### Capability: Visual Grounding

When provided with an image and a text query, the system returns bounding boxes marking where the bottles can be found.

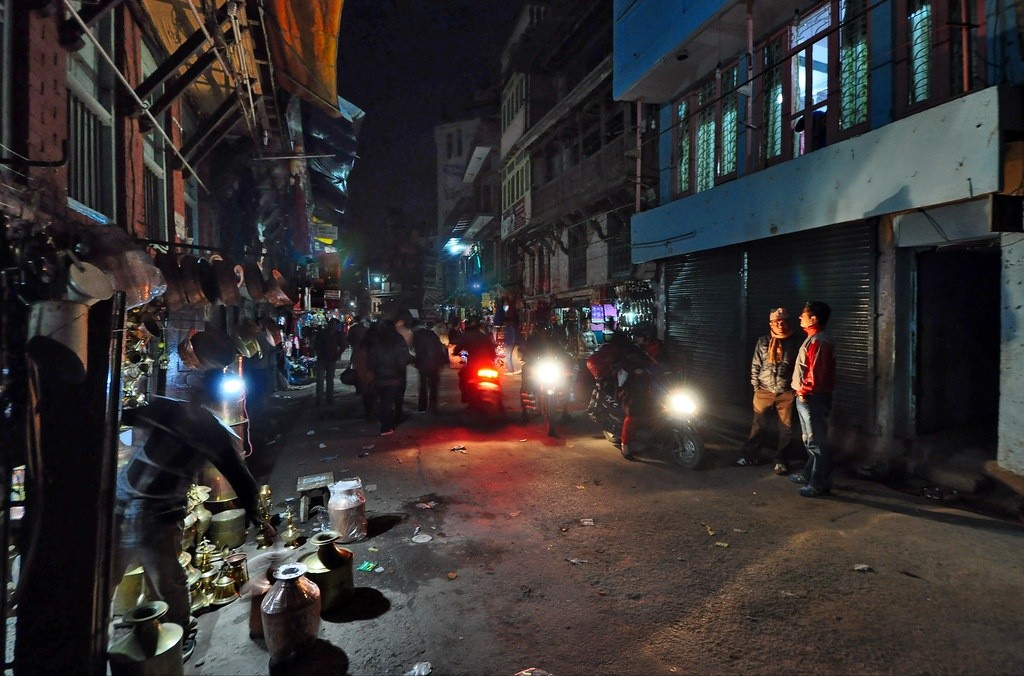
[108,478,365,676]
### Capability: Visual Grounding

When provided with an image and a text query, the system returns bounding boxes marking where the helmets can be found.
[631,321,657,344]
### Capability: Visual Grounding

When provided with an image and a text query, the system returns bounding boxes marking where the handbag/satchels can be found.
[340,360,357,384]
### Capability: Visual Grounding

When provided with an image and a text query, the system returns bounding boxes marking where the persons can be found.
[517,321,577,451]
[425,306,518,415]
[314,316,348,406]
[499,317,522,374]
[110,365,275,665]
[789,299,838,497]
[412,320,448,421]
[737,307,803,474]
[613,318,676,461]
[576,317,605,383]
[345,312,413,445]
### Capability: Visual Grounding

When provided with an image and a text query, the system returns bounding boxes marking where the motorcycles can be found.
[518,342,578,437]
[588,359,704,470]
[453,354,499,431]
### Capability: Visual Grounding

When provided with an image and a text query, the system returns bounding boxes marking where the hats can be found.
[770,307,789,320]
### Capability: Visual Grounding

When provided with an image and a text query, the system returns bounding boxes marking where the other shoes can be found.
[380,429,393,436]
[418,410,426,414]
[182,639,194,661]
[789,473,809,484]
[621,442,633,460]
[737,456,758,466]
[797,485,830,496]
[774,463,787,474]
